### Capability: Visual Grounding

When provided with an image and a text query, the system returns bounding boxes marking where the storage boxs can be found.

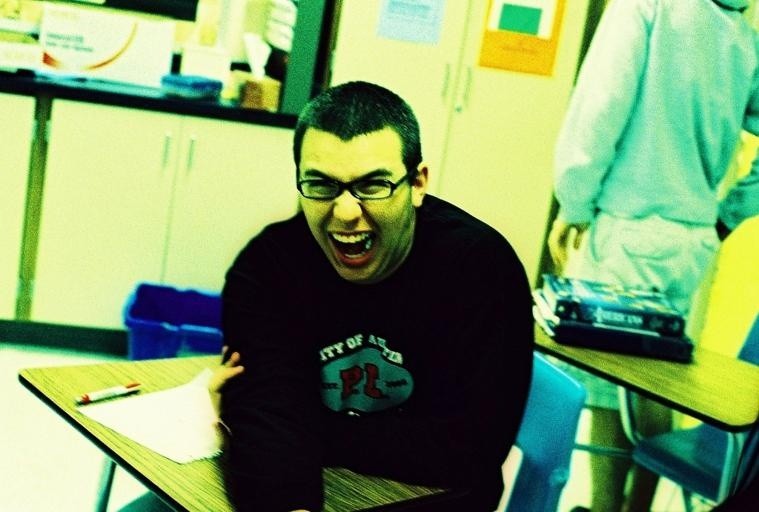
[122,280,224,361]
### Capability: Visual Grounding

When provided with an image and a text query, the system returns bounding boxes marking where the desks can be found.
[532,328,759,433]
[19,353,468,512]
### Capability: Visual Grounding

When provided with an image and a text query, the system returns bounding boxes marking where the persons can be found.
[540,1,759,512]
[118,76,538,512]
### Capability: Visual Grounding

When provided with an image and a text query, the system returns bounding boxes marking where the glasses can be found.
[296,167,410,201]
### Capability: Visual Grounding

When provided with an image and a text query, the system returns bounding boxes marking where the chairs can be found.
[498,349,587,512]
[620,312,759,512]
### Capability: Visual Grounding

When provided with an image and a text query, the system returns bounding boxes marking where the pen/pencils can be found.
[75,384,140,404]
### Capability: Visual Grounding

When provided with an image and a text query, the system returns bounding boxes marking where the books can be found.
[535,272,694,367]
